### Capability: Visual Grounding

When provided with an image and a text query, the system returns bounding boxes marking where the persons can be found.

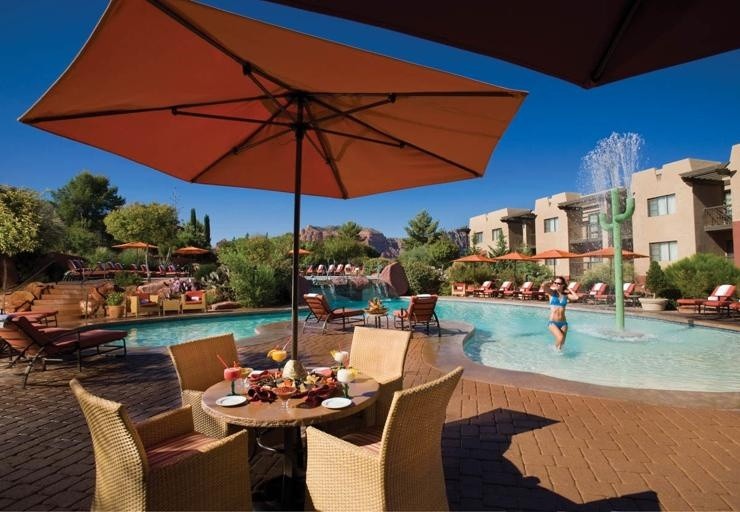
[180,280,192,301]
[540,276,580,351]
[169,276,181,295]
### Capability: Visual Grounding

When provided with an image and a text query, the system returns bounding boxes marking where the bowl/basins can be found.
[363,308,390,314]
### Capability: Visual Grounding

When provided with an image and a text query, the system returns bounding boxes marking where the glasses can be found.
[554,282,564,285]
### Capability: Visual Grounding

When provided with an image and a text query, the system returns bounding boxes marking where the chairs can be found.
[701,284,737,318]
[167,332,256,461]
[451,281,468,297]
[314,325,412,438]
[392,294,441,337]
[305,263,351,276]
[472,281,635,307]
[124,289,208,319]
[302,293,365,336]
[68,378,254,511]
[302,365,464,511]
[0,309,126,388]
[62,260,189,282]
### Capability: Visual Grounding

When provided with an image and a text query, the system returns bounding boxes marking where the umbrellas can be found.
[450,253,499,282]
[574,246,650,284]
[491,251,541,282]
[530,248,582,280]
[111,241,159,269]
[266,0,740,90]
[15,0,530,360]
[176,245,209,263]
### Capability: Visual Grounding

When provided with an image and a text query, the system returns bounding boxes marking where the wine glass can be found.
[238,366,251,395]
[271,351,288,373]
[273,387,297,413]
[333,350,350,367]
[223,367,241,395]
[337,369,354,399]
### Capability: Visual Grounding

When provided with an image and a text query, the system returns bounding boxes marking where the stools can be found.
[676,298,706,314]
[728,301,740,322]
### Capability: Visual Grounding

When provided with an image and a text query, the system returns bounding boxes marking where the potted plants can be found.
[105,291,125,319]
[206,289,223,309]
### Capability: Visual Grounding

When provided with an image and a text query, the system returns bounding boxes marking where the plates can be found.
[322,397,353,409]
[216,395,247,407]
